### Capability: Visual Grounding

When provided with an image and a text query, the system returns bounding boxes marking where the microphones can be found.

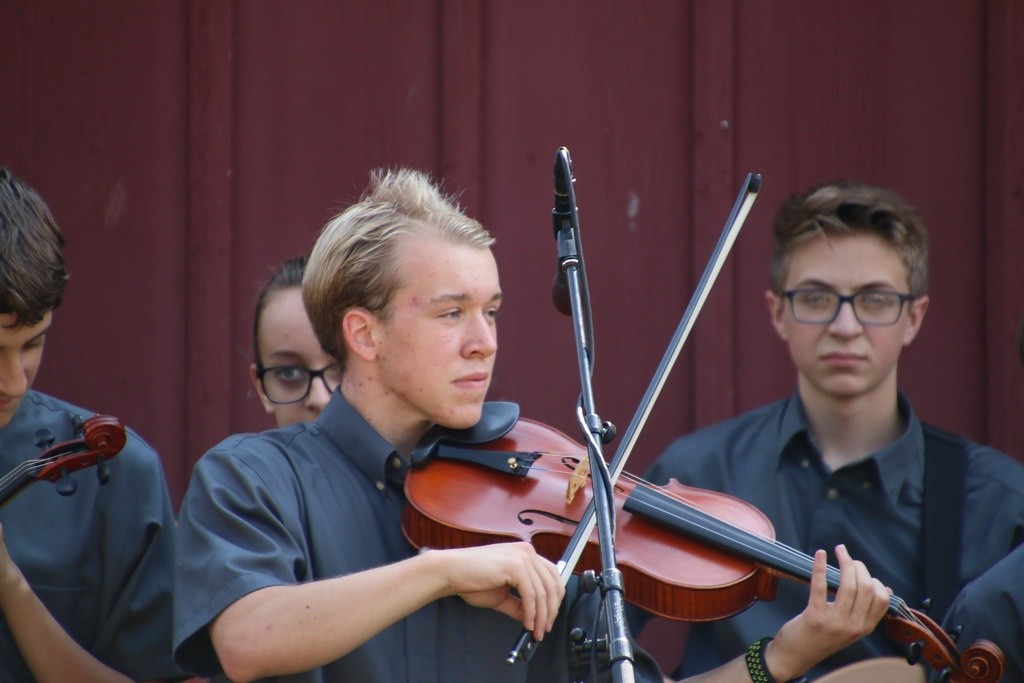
[552,148,590,315]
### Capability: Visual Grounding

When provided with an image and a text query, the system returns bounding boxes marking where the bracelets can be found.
[747,636,807,682]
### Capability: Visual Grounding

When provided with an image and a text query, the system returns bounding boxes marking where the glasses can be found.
[776,288,916,325]
[256,359,347,404]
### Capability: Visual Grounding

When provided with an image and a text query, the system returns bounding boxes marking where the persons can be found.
[169,167,894,683]
[0,170,174,683]
[616,187,1024,683]
[249,252,342,431]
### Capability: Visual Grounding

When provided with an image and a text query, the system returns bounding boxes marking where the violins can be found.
[399,413,1007,683]
[0,414,130,511]
